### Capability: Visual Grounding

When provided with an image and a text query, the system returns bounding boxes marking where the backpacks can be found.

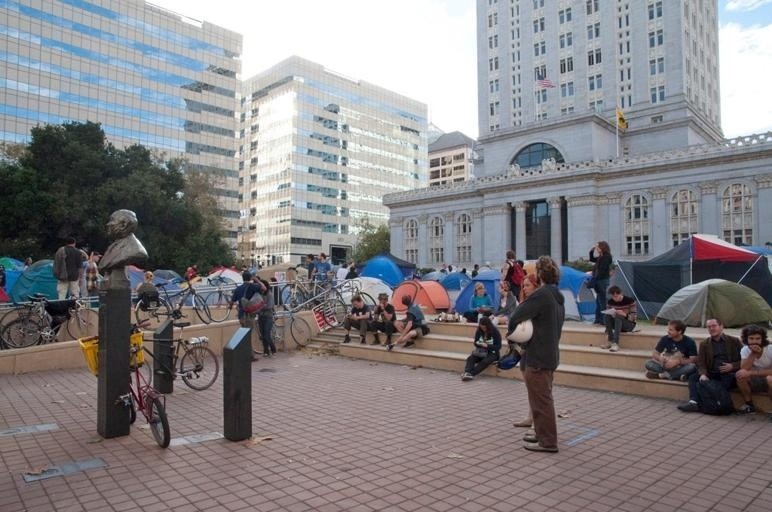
[696,377,737,416]
[53,246,68,281]
[508,258,525,285]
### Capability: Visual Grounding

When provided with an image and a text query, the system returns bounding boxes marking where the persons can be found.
[676,316,738,415]
[642,317,697,380]
[733,323,772,414]
[503,253,567,455]
[458,314,503,382]
[94,207,152,271]
[510,272,542,434]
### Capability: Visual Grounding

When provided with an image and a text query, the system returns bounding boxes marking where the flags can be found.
[536,72,556,91]
[615,107,630,131]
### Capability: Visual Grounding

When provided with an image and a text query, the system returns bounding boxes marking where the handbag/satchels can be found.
[471,345,489,358]
[240,284,266,313]
[422,323,430,335]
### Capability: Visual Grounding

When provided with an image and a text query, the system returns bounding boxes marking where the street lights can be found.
[241,253,262,268]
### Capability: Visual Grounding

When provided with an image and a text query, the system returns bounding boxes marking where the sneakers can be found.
[385,343,394,351]
[252,353,278,362]
[599,342,619,351]
[343,336,351,344]
[679,375,690,381]
[360,337,366,344]
[402,341,416,349]
[460,371,474,381]
[735,401,757,415]
[525,429,536,435]
[522,435,538,443]
[646,371,661,379]
[513,419,532,427]
[523,441,559,452]
[369,339,381,345]
[676,402,700,412]
[382,339,391,346]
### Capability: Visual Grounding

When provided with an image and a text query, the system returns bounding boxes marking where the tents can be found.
[607,232,772,321]
[651,278,772,331]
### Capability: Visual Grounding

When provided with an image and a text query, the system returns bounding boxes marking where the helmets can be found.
[505,318,534,343]
[496,347,523,371]
